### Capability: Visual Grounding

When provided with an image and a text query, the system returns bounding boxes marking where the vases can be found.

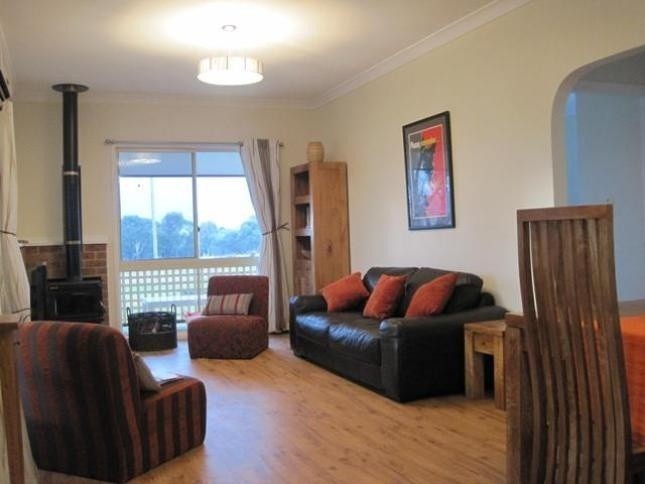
[307,142,324,163]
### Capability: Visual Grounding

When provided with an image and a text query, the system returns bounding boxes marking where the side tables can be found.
[463,319,507,409]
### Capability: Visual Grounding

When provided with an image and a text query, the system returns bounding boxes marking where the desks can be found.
[506,314,645,483]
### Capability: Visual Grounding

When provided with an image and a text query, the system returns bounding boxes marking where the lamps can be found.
[196,24,263,86]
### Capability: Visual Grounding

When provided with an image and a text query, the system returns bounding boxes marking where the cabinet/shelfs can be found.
[289,161,350,296]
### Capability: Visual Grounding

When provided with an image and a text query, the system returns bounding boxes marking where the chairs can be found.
[188,276,268,359]
[516,205,631,483]
[14,321,206,484]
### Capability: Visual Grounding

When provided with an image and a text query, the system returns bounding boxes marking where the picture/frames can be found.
[402,111,455,230]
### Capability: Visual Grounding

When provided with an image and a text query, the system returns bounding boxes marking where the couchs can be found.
[290,267,507,405]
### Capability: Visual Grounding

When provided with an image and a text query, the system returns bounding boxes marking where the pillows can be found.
[201,293,253,316]
[364,274,407,319]
[319,272,370,313]
[404,273,457,317]
[131,352,159,394]
